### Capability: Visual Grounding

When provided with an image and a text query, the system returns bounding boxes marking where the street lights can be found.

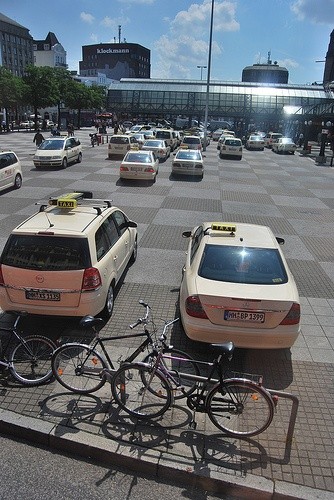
[197,66,207,80]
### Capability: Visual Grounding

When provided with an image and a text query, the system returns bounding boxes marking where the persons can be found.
[285,126,304,147]
[2,118,126,147]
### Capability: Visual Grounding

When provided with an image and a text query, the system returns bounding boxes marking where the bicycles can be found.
[0,309,60,387]
[50,299,200,400]
[113,318,278,438]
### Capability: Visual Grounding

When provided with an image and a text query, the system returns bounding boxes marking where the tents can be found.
[95,113,112,117]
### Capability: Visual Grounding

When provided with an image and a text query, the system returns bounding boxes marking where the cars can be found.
[0,150,23,191]
[195,128,283,151]
[272,138,296,154]
[123,120,183,152]
[9,120,58,129]
[180,136,203,153]
[32,135,83,170]
[219,138,243,159]
[142,140,171,161]
[0,190,138,318]
[120,147,159,183]
[178,221,301,349]
[172,149,206,178]
[108,134,139,159]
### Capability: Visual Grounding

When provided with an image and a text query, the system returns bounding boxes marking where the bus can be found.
[93,113,113,124]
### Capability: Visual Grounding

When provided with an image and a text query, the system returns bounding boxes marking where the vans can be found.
[176,119,232,130]
